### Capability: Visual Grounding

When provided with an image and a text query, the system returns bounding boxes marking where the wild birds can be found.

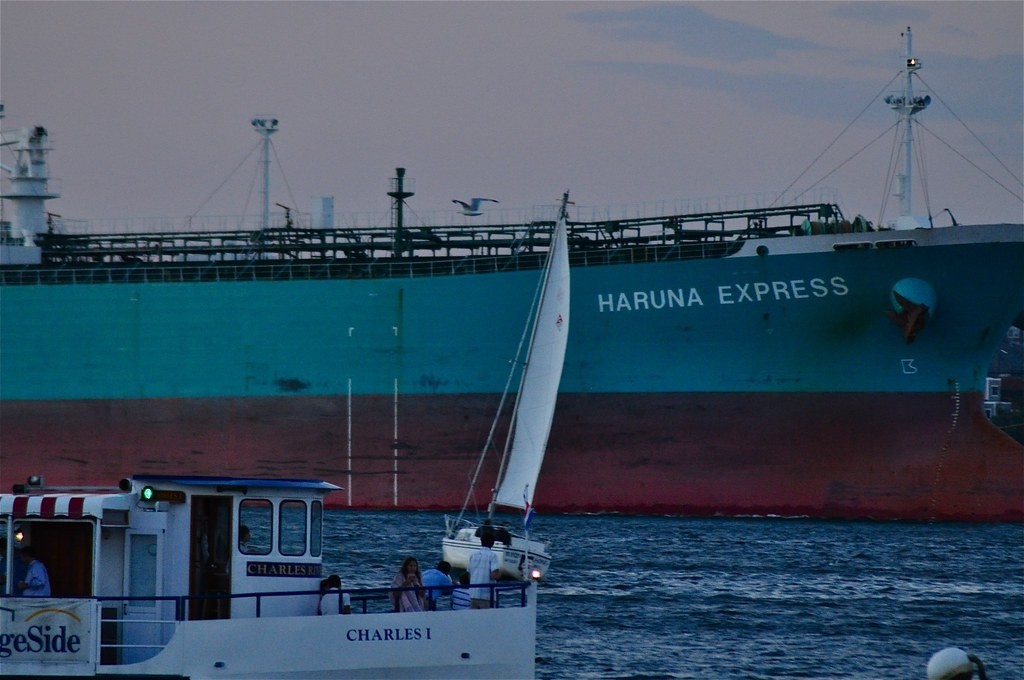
[452,197,501,216]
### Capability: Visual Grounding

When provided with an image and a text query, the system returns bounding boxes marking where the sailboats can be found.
[441,190,576,580]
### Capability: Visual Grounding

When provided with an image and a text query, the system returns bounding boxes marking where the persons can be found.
[420,561,453,611]
[226,524,255,573]
[451,572,471,611]
[317,575,351,616]
[0,537,52,596]
[387,557,425,613]
[466,531,501,609]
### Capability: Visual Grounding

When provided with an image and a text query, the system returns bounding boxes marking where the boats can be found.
[0,474,537,680]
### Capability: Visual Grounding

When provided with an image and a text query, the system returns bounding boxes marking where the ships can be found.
[0,24,1024,525]
